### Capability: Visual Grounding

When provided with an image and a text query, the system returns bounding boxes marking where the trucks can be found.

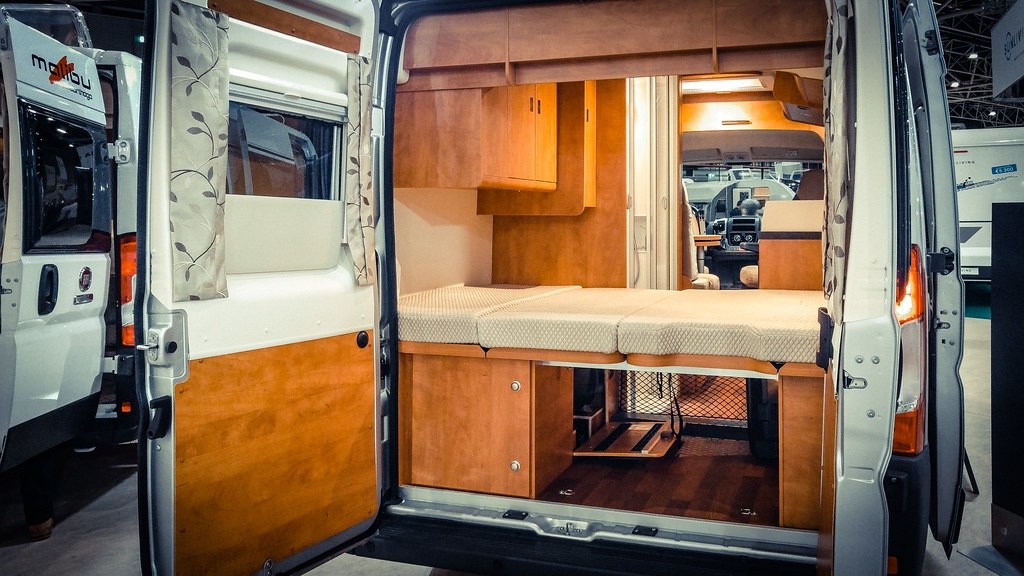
[1,8,320,476]
[127,1,965,576]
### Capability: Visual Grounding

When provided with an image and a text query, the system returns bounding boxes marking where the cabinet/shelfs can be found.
[398,354,573,499]
[393,83,557,192]
[477,80,596,217]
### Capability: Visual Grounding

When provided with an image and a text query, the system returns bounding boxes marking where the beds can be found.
[397,282,828,364]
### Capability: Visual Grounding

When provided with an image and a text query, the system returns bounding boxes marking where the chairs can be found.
[740,169,824,288]
[682,179,721,289]
